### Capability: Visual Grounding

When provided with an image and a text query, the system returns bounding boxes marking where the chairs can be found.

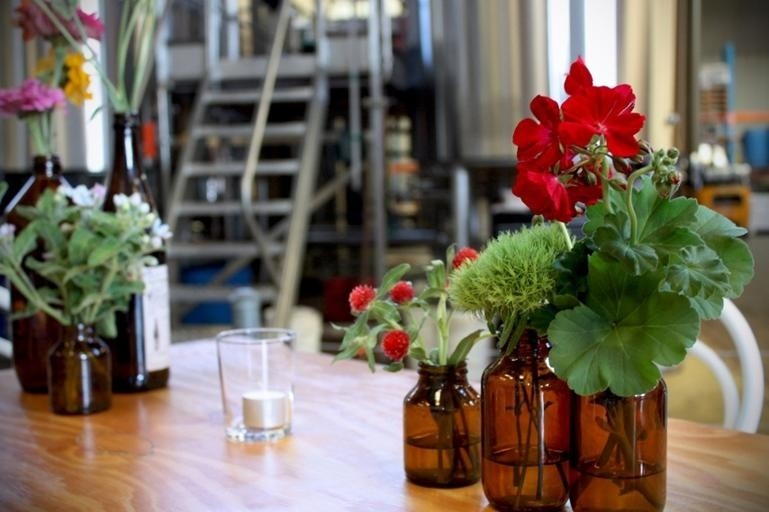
[657,292,765,432]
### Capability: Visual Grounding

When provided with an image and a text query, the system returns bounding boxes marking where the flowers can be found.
[338,244,493,472]
[1,177,176,398]
[450,224,566,497]
[512,61,757,505]
[1,1,105,155]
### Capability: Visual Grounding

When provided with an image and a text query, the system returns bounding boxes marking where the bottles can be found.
[571,376,669,510]
[47,323,114,416]
[404,359,483,487]
[101,112,174,390]
[4,154,83,398]
[483,327,570,512]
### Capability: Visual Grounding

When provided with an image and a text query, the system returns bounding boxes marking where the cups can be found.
[215,328,298,443]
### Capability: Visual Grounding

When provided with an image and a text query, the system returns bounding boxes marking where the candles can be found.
[243,391,287,428]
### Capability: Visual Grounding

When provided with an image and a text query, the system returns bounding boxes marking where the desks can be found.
[4,328,768,509]
